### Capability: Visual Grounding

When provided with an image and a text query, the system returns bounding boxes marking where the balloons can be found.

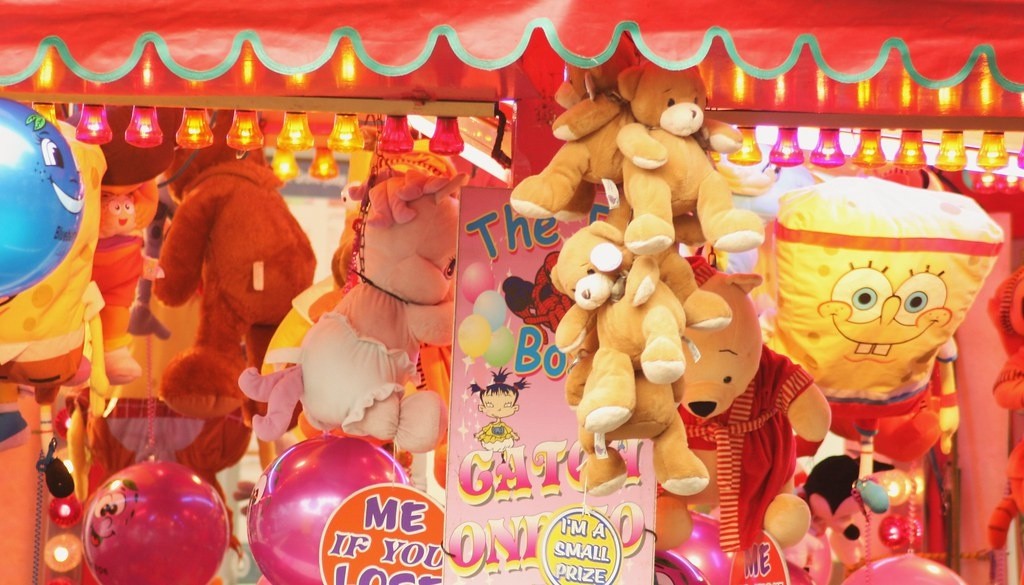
[83,458,229,585]
[246,436,407,585]
[0,97,81,298]
[652,512,733,584]
[838,554,965,585]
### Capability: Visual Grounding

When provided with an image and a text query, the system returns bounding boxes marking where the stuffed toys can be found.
[774,177,1003,458]
[795,456,895,540]
[988,266,1024,552]
[0,107,471,550]
[512,30,763,495]
[656,267,832,542]
